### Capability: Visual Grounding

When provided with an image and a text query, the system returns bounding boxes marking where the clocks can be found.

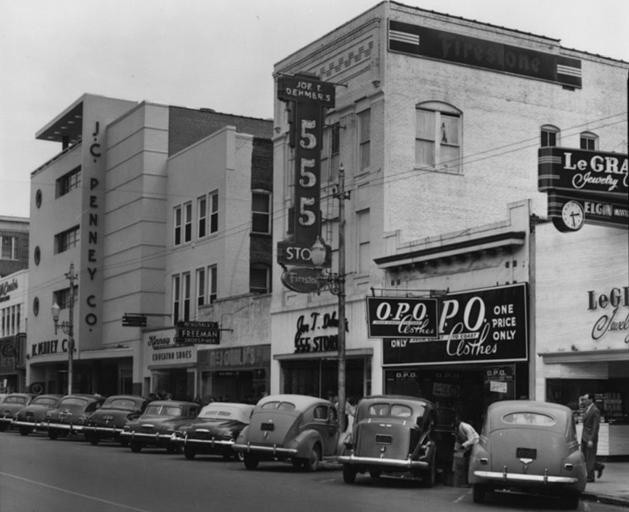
[554,200,585,233]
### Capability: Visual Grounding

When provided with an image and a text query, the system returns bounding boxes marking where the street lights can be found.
[309,232,350,435]
[50,302,74,395]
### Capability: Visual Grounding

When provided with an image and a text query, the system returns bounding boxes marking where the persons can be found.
[579,393,605,483]
[330,395,357,435]
[140,389,173,413]
[445,415,479,488]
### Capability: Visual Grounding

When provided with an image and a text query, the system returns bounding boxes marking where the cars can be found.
[121,397,205,455]
[183,400,257,461]
[0,388,144,449]
[231,394,344,470]
[336,393,456,485]
[469,398,587,507]
